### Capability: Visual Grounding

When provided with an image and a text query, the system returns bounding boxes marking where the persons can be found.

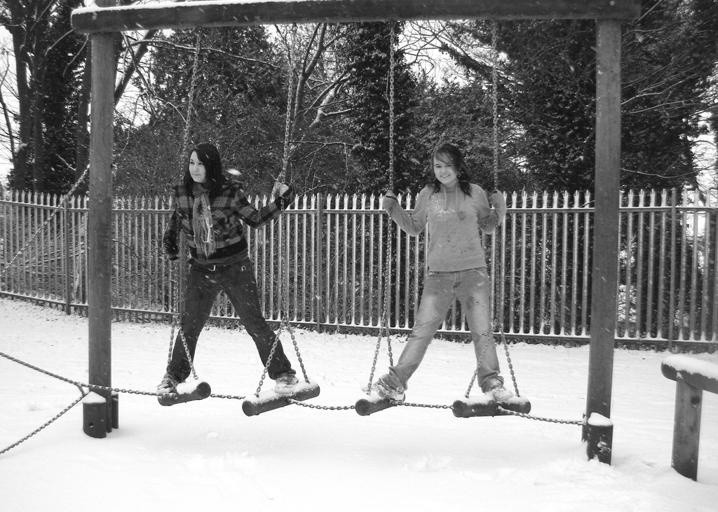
[155,142,299,394]
[373,142,514,402]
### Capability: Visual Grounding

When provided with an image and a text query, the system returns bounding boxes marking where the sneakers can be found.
[361,379,407,404]
[274,372,299,396]
[484,384,516,404]
[155,373,184,395]
